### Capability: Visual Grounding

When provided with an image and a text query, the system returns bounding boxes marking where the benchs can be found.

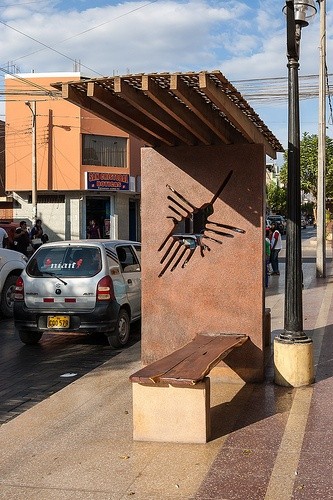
[131,333,247,444]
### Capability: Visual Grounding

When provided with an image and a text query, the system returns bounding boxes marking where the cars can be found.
[301,216,308,228]
[14,239,143,348]
[265,214,287,236]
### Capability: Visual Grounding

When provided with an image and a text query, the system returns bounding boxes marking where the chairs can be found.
[67,251,93,272]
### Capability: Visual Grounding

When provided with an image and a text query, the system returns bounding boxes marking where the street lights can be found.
[272,0,319,388]
[25,99,38,227]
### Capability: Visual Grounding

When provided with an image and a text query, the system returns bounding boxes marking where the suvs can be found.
[0,249,28,319]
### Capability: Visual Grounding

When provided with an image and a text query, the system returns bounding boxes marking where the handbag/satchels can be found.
[31,235,43,247]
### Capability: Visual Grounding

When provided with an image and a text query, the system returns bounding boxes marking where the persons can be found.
[13,221,30,258]
[87,219,101,239]
[265,219,287,288]
[0,227,8,248]
[31,219,47,255]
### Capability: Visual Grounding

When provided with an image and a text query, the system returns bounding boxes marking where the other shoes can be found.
[271,271,280,275]
